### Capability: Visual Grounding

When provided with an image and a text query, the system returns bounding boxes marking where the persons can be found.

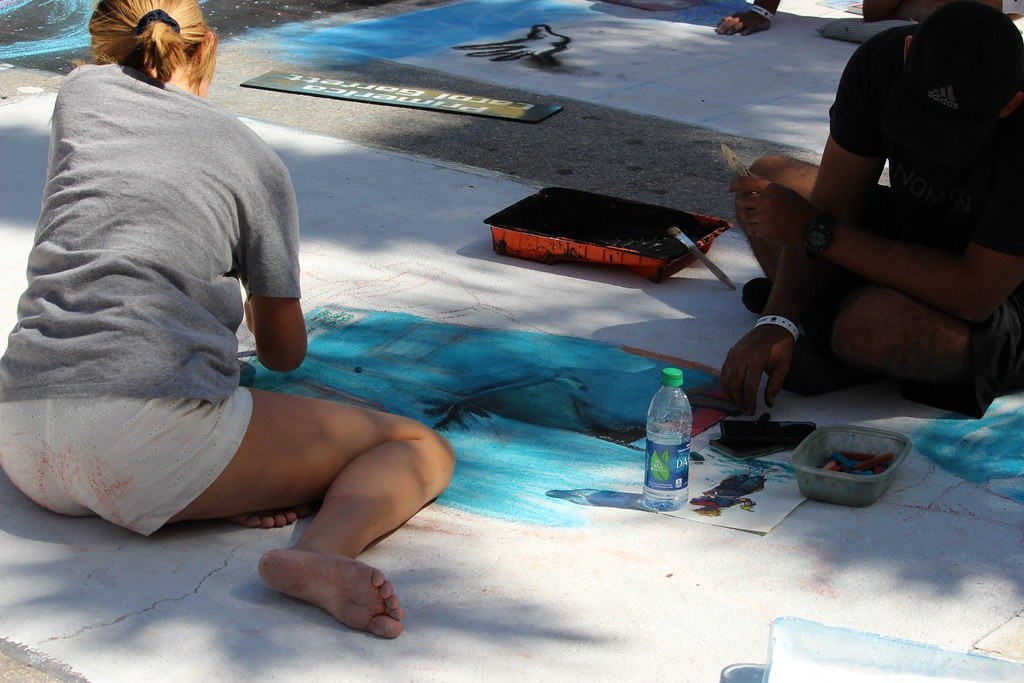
[1,0,459,641]
[714,0,1024,35]
[718,0,1024,419]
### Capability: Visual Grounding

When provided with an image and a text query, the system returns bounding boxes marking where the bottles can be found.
[643,367,693,511]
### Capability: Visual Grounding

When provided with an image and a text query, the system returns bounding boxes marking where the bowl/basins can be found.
[791,424,911,507]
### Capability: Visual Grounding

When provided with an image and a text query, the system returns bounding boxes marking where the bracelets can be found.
[755,313,800,344]
[751,4,776,22]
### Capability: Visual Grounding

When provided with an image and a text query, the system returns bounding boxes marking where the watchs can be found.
[801,210,838,259]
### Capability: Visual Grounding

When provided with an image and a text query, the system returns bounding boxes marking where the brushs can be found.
[668,225,737,288]
